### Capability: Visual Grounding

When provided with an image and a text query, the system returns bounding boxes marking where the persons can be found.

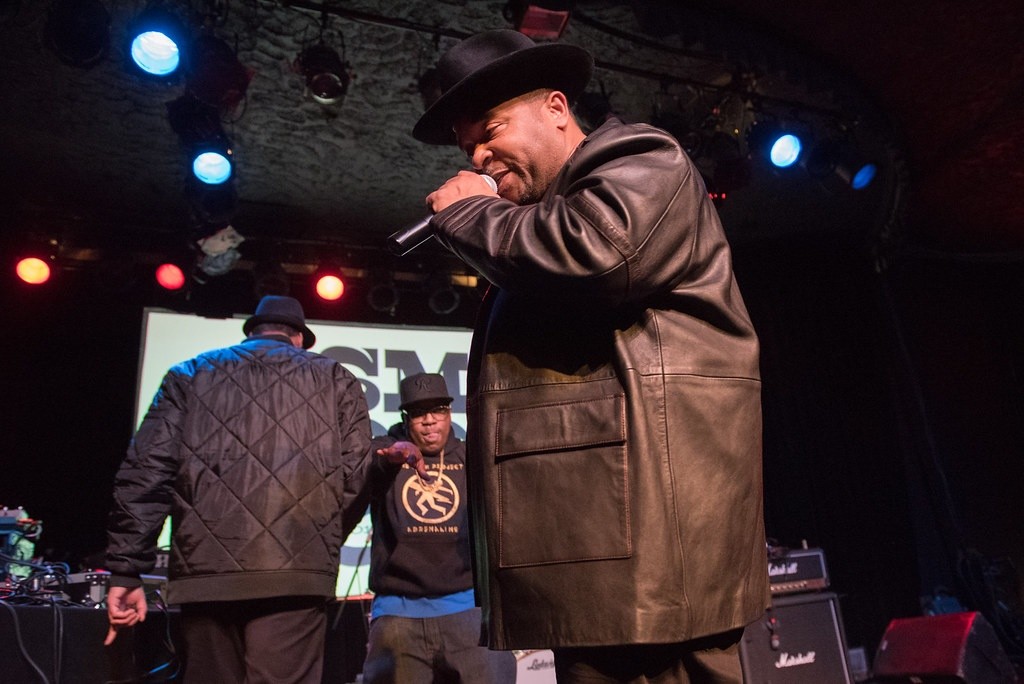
[98,296,376,683]
[426,39,770,684]
[360,381,519,681]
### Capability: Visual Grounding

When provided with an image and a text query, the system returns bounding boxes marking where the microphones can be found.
[366,528,373,543]
[387,174,498,255]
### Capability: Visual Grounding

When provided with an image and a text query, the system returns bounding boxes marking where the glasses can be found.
[402,402,452,424]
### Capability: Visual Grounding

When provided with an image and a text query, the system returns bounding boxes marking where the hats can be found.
[398,372,454,411]
[411,29,594,147]
[242,295,317,350]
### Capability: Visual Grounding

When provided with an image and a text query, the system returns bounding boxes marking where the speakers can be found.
[735,590,1022,684]
